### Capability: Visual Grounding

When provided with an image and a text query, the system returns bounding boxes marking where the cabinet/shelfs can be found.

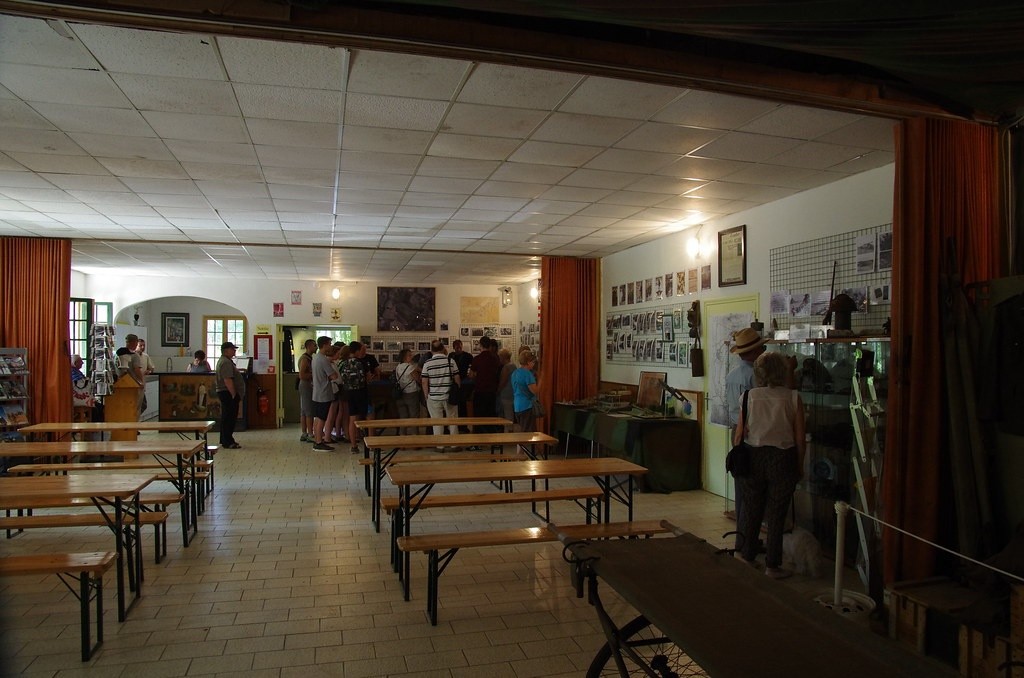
[764,337,890,572]
[0,347,34,477]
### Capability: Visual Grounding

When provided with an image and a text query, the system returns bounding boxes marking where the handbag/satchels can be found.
[447,383,459,405]
[295,377,300,389]
[726,445,750,479]
[532,400,545,417]
[72,379,96,408]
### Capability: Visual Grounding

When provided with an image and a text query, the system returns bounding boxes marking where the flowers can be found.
[134,304,144,314]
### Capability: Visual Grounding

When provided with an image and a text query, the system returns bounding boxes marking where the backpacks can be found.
[392,365,415,399]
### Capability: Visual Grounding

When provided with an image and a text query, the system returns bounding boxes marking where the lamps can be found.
[331,284,343,300]
[499,287,515,306]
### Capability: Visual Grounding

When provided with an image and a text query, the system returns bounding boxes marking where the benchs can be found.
[0,511,169,592]
[7,459,218,515]
[379,488,604,571]
[156,472,209,528]
[0,552,118,662]
[0,493,185,565]
[395,520,675,626]
[357,454,529,493]
[154,472,210,517]
[205,442,222,491]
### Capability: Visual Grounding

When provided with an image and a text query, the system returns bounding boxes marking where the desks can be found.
[364,432,558,532]
[0,439,204,549]
[0,474,159,622]
[597,407,701,493]
[552,398,632,459]
[354,417,512,496]
[384,457,648,602]
[16,421,217,494]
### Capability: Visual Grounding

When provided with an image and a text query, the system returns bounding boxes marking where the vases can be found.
[134,314,140,325]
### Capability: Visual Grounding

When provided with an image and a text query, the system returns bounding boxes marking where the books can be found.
[0,355,31,476]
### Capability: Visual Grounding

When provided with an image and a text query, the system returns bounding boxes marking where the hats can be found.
[221,342,239,349]
[729,328,770,354]
[126,334,141,342]
[116,348,136,355]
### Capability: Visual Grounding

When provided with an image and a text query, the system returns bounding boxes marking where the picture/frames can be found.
[359,286,540,369]
[668,343,677,366]
[606,223,747,369]
[636,371,666,410]
[159,311,190,348]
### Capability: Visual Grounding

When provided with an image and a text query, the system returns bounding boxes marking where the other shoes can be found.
[495,446,500,449]
[222,442,241,449]
[435,448,445,453]
[452,447,463,452]
[766,566,791,579]
[760,547,768,553]
[300,432,362,453]
[466,446,483,451]
[743,559,760,568]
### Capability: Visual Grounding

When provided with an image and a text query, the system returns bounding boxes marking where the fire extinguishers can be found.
[255,383,271,416]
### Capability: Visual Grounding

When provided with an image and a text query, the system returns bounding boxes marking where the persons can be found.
[187,350,211,373]
[114,334,143,382]
[134,339,155,435]
[727,327,771,551]
[215,342,245,449]
[735,352,807,579]
[71,355,104,424]
[298,335,541,462]
